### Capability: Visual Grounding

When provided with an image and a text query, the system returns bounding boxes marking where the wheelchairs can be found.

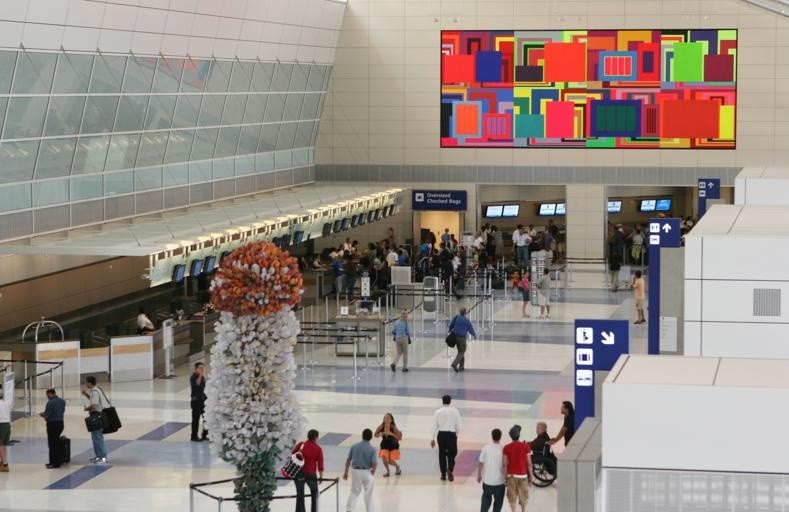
[529,439,559,487]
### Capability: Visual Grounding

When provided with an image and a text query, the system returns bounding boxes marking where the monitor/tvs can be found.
[323,204,397,238]
[608,200,622,213]
[486,204,520,218]
[173,231,305,283]
[539,203,566,216]
[640,199,672,212]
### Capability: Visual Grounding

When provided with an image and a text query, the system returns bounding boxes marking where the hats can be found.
[509,425,522,438]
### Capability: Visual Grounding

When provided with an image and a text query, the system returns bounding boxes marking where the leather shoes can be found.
[45,463,61,469]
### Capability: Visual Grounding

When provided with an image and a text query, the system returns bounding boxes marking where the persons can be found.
[82,376,110,464]
[0,389,11,472]
[343,395,574,512]
[190,362,209,442]
[292,429,324,512]
[137,304,155,334]
[448,307,477,373]
[390,308,411,372]
[39,388,66,468]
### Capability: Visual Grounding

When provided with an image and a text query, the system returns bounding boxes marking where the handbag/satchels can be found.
[277,440,305,487]
[84,410,110,433]
[542,443,558,476]
[408,335,412,344]
[97,406,123,435]
[445,331,459,349]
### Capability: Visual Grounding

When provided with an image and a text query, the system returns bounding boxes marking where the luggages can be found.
[58,435,72,465]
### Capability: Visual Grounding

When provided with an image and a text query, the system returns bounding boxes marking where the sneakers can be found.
[391,364,397,373]
[450,363,465,373]
[456,295,465,301]
[521,314,552,319]
[88,455,110,465]
[395,469,402,476]
[633,318,646,324]
[401,368,409,372]
[191,437,210,442]
[382,472,391,477]
[0,460,10,473]
[440,468,454,482]
